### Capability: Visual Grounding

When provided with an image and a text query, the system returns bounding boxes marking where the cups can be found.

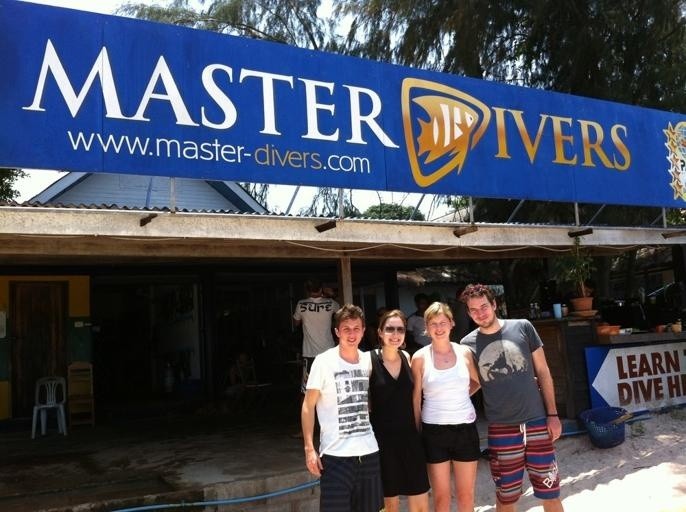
[673,324,681,333]
[553,304,562,318]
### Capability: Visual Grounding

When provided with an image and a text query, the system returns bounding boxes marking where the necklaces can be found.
[444,359,450,363]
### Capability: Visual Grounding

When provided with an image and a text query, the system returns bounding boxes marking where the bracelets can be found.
[547,412,559,417]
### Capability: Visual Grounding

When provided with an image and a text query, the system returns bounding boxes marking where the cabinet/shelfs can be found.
[532,315,601,421]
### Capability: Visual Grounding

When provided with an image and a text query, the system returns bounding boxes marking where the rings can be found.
[558,433,562,435]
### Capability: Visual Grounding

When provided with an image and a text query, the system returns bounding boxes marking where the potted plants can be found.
[547,249,598,316]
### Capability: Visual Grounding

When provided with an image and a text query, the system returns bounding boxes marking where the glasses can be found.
[381,326,406,334]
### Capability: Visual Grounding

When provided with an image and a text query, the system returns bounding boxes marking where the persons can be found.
[291,276,342,440]
[459,282,566,512]
[411,301,543,511]
[300,303,385,512]
[406,294,433,353]
[363,309,432,512]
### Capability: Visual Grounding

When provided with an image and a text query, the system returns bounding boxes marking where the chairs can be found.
[30,360,94,438]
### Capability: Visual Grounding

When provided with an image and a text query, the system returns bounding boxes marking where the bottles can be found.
[676,319,682,332]
[562,304,569,317]
[530,303,549,318]
